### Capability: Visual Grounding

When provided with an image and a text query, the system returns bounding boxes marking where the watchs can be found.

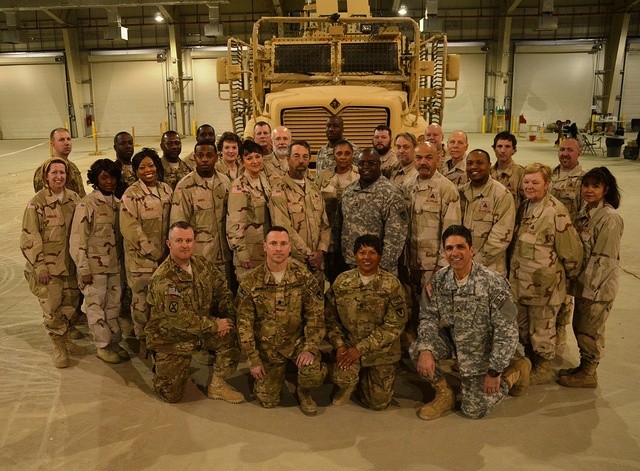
[488,368,501,377]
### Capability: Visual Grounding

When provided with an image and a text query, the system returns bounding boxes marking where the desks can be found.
[601,135,627,157]
[493,114,515,133]
[591,114,626,134]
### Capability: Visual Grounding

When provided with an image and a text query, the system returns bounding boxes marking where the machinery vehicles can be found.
[216,0,460,177]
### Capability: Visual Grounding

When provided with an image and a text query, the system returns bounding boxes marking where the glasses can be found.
[357,160,380,166]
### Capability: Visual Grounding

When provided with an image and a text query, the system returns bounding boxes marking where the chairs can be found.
[583,135,597,156]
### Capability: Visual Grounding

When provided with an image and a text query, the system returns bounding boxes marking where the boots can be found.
[64,338,85,355]
[54,337,68,368]
[419,378,455,420]
[501,357,532,397]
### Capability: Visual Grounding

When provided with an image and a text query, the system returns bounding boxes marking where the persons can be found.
[424,122,449,175]
[315,140,360,285]
[119,147,173,359]
[385,131,418,187]
[507,162,584,385]
[69,158,129,364]
[452,148,516,372]
[182,124,220,171]
[267,140,331,292]
[160,130,193,190]
[254,121,272,155]
[34,127,87,326]
[402,140,461,323]
[338,149,410,278]
[114,131,137,198]
[214,132,244,180]
[316,115,361,179]
[225,141,272,283]
[489,131,525,210]
[262,125,316,189]
[372,124,398,167]
[19,156,82,369]
[324,234,408,411]
[234,226,328,416]
[436,128,471,187]
[169,139,231,355]
[546,138,587,348]
[408,224,532,420]
[558,166,624,388]
[144,221,245,404]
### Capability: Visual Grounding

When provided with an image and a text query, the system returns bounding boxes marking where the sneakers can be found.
[558,367,598,380]
[297,386,317,416]
[332,386,353,406]
[559,372,597,388]
[208,383,244,404]
[110,343,130,361]
[119,315,135,337]
[529,355,554,376]
[193,349,214,366]
[71,310,87,325]
[139,340,148,358]
[97,347,120,364]
[66,327,82,339]
